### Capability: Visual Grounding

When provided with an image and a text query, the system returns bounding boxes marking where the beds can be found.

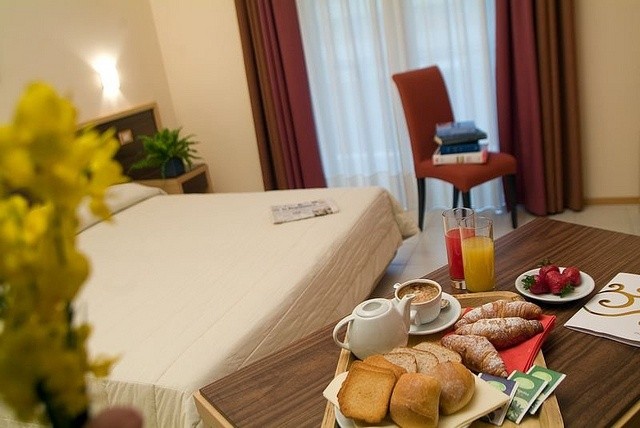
[69,182,421,428]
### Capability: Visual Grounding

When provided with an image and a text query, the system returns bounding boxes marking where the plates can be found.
[334,405,472,428]
[391,291,462,336]
[514,266,596,304]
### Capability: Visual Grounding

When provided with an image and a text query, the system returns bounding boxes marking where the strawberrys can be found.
[524,261,581,298]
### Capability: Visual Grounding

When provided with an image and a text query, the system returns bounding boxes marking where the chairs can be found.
[392,66,519,232]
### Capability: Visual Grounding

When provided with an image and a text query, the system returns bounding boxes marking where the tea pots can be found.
[332,294,416,360]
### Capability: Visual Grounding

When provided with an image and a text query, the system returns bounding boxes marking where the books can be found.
[431,121,489,165]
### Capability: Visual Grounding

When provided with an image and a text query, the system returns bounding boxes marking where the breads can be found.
[337,342,475,428]
[440,299,545,378]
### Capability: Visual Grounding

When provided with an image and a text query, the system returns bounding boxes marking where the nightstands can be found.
[75,102,213,194]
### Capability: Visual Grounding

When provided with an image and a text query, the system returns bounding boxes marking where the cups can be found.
[459,216,496,293]
[441,207,475,291]
[394,279,442,327]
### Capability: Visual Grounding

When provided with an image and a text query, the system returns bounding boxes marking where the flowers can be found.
[0,83,125,428]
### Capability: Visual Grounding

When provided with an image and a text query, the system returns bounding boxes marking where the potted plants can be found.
[127,127,203,178]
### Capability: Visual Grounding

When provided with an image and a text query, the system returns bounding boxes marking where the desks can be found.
[193,217,640,428]
